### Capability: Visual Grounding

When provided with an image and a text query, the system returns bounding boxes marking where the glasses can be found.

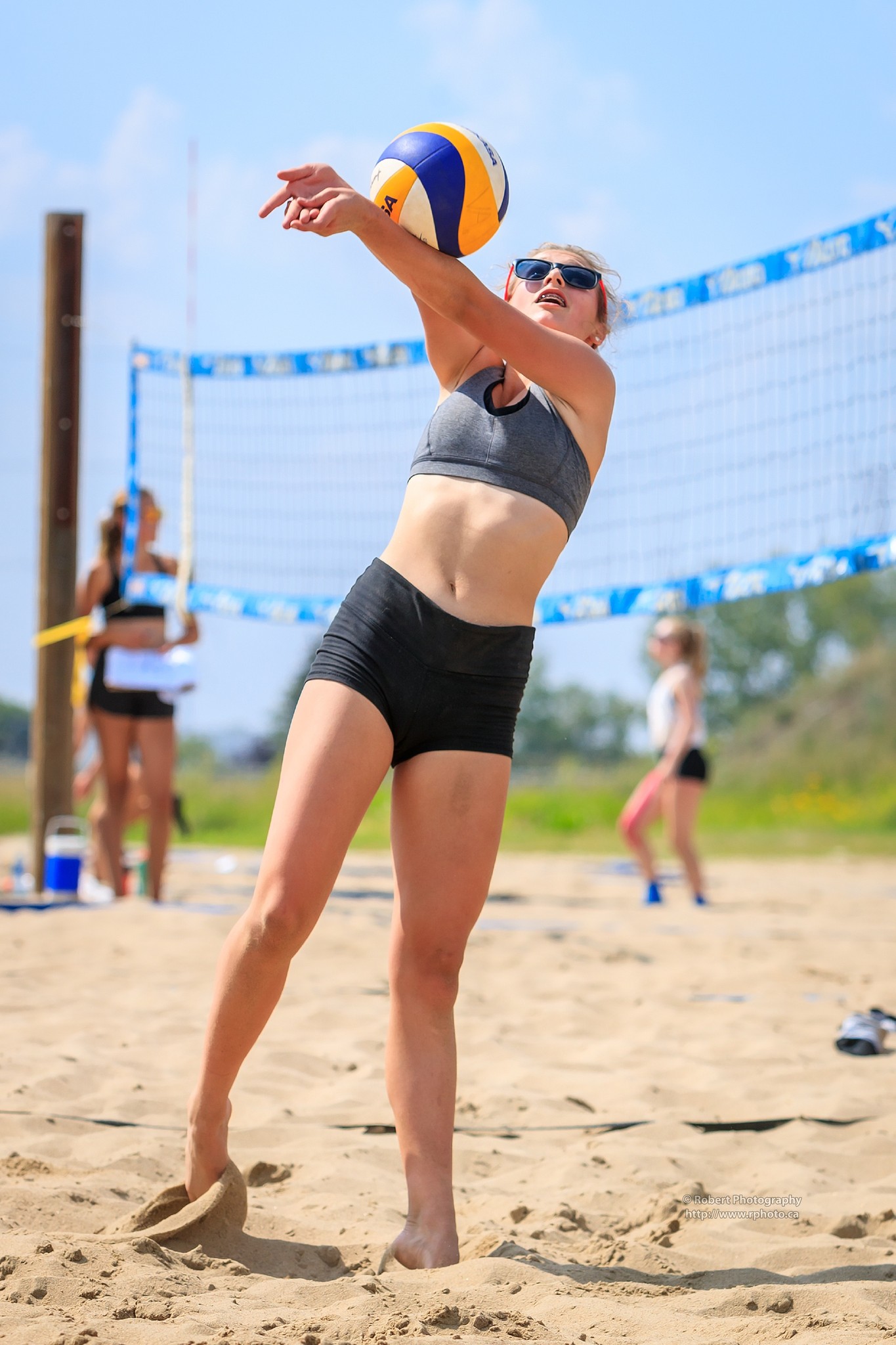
[504,258,607,322]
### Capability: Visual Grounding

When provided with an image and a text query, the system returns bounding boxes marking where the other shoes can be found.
[645,882,663,904]
[695,894,706,905]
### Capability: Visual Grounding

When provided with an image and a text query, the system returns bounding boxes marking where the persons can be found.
[181,162,629,1266]
[615,617,709,909]
[73,486,197,902]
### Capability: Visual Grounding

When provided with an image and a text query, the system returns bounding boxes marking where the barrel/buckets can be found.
[42,816,89,896]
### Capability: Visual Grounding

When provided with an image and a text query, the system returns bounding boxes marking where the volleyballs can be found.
[369,122,509,259]
